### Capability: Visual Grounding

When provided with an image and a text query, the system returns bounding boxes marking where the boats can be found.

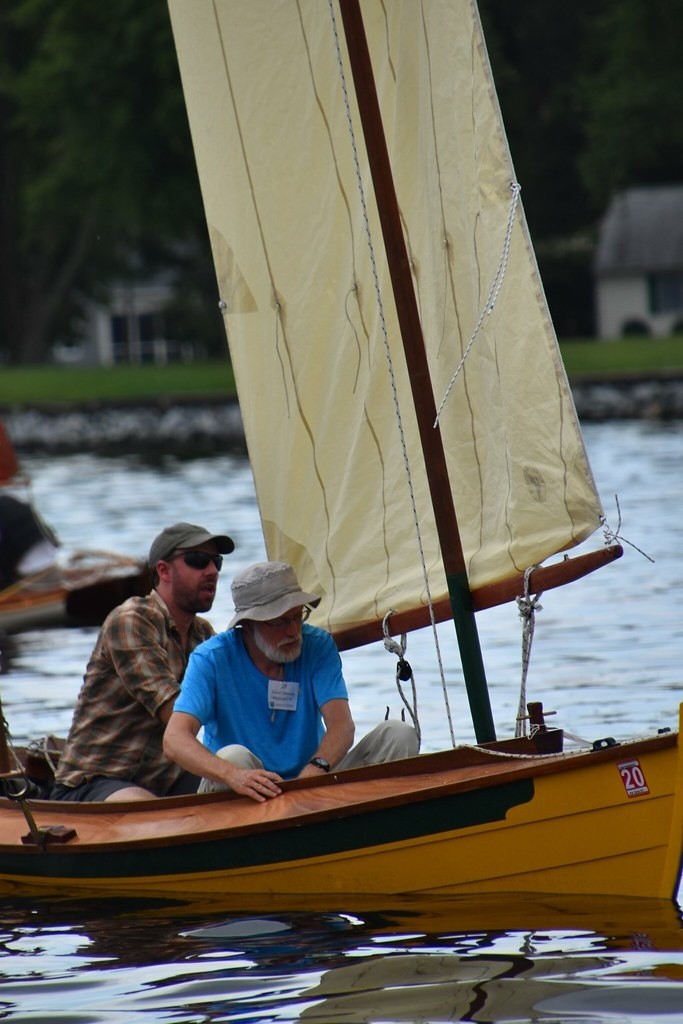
[1,549,162,638]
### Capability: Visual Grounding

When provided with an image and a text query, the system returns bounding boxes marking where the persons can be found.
[54,521,235,804]
[162,561,419,803]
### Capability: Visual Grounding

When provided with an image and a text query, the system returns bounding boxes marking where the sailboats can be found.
[0,0,682,915]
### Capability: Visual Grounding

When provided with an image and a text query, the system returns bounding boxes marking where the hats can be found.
[227,562,321,629]
[149,522,234,574]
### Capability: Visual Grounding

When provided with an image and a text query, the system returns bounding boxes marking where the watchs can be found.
[308,757,330,772]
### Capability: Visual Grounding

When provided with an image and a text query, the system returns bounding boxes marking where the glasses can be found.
[168,550,223,571]
[263,604,312,629]
